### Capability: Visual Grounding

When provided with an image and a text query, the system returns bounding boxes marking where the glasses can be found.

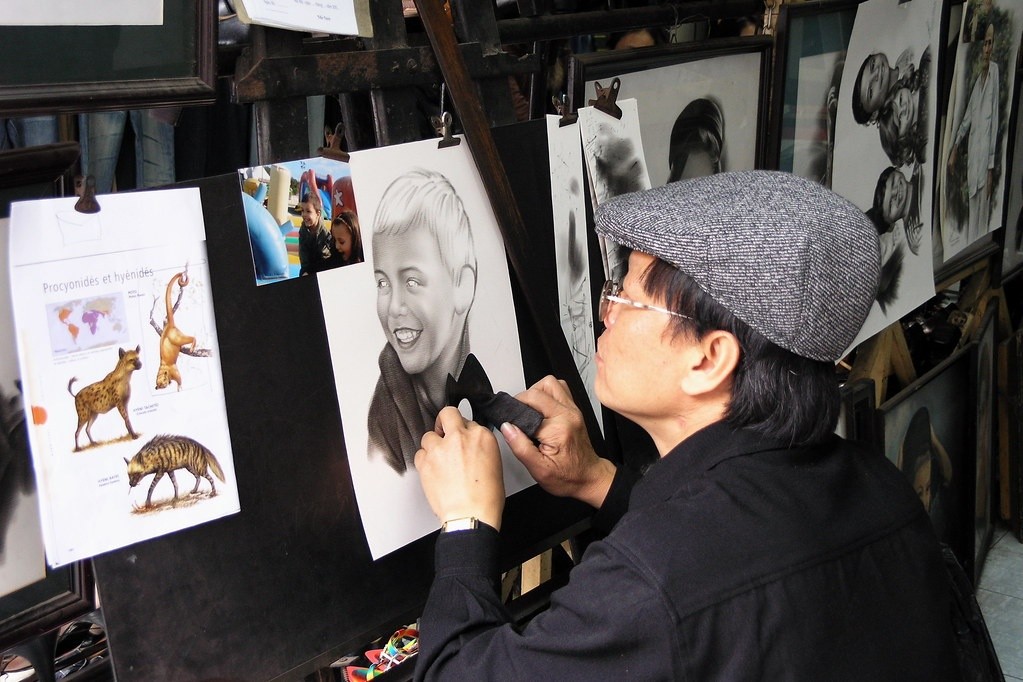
[600,280,693,322]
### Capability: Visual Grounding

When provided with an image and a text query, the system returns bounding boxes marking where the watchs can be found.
[441,517,499,538]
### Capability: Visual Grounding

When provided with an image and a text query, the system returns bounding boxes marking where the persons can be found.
[298,193,365,277]
[414,170,1006,682]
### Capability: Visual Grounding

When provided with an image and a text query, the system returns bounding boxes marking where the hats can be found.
[594,170,880,361]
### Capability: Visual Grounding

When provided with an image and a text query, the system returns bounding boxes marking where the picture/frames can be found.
[0,3,217,121]
[933,0,1010,287]
[562,37,772,188]
